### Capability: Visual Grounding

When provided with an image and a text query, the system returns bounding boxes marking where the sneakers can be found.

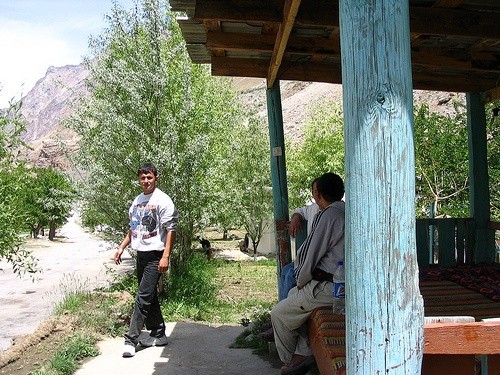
[122,345,135,356]
[139,335,169,347]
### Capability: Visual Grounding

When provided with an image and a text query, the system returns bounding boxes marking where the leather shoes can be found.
[257,321,275,341]
[281,354,317,375]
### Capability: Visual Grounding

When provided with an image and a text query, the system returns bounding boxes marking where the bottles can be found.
[333,261,346,316]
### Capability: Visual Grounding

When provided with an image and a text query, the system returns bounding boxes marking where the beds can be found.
[307,263,500,375]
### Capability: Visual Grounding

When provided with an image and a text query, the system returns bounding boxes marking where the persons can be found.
[270,172,346,375]
[114,161,179,358]
[290,178,324,238]
